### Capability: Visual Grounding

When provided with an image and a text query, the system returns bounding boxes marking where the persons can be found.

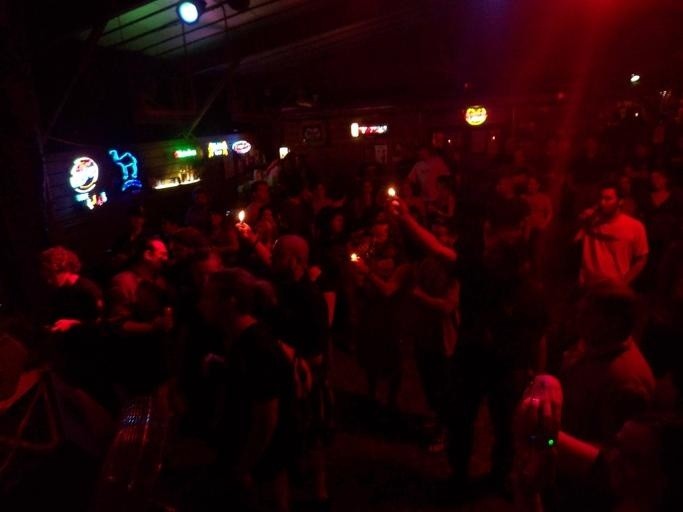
[1,122,683,512]
